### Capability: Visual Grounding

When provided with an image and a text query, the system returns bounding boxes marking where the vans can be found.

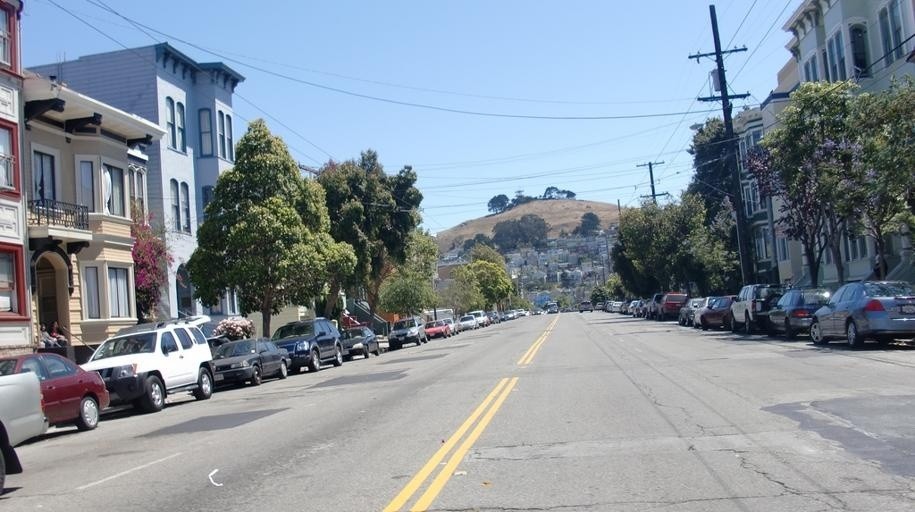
[546,302,558,314]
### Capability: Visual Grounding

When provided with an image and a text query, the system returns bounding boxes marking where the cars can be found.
[578,300,594,313]
[209,334,293,390]
[422,306,545,343]
[338,325,382,362]
[594,290,735,331]
[766,285,832,339]
[0,349,114,436]
[805,280,915,348]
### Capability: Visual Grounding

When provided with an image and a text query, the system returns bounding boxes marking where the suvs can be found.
[79,313,218,417]
[386,315,429,349]
[270,314,345,374]
[728,281,792,335]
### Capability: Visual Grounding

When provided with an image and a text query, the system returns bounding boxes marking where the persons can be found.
[875,251,883,281]
[48,320,68,346]
[39,319,58,348]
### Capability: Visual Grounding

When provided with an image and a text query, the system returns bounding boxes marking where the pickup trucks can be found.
[0,368,55,495]
